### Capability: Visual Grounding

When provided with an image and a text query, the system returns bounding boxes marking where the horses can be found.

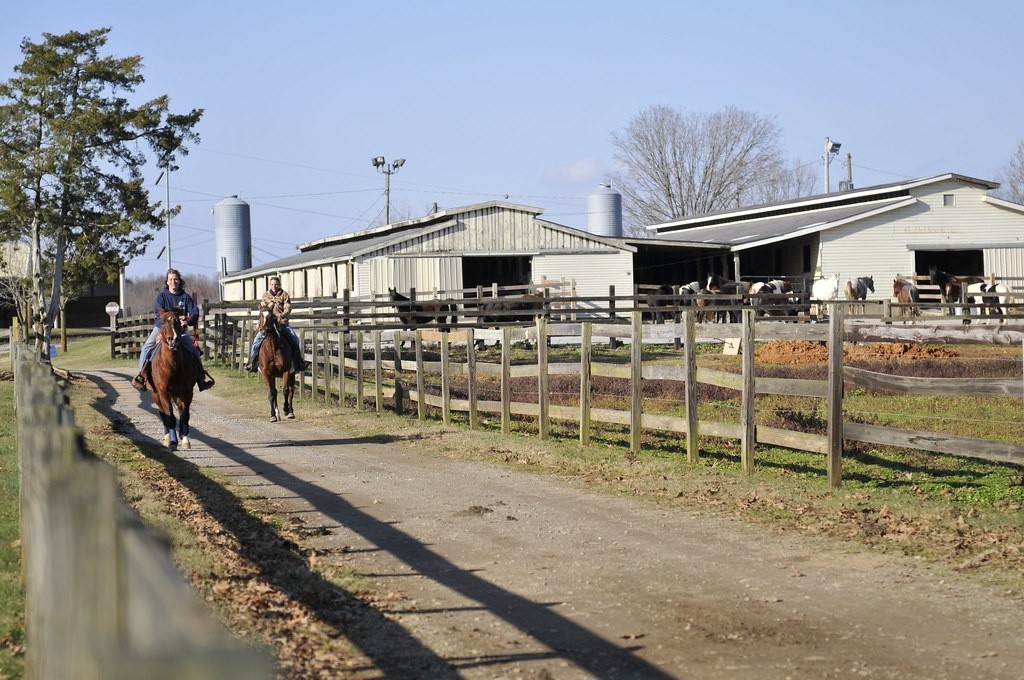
[148,306,196,451]
[811,264,1015,324]
[484,290,545,322]
[648,284,673,324]
[679,273,794,322]
[257,301,297,422]
[388,286,457,332]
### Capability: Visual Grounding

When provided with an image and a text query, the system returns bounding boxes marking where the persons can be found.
[245,277,308,371]
[132,269,215,392]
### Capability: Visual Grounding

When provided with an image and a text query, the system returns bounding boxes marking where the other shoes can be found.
[198,378,212,392]
[297,361,308,371]
[141,384,147,391]
[245,364,258,373]
